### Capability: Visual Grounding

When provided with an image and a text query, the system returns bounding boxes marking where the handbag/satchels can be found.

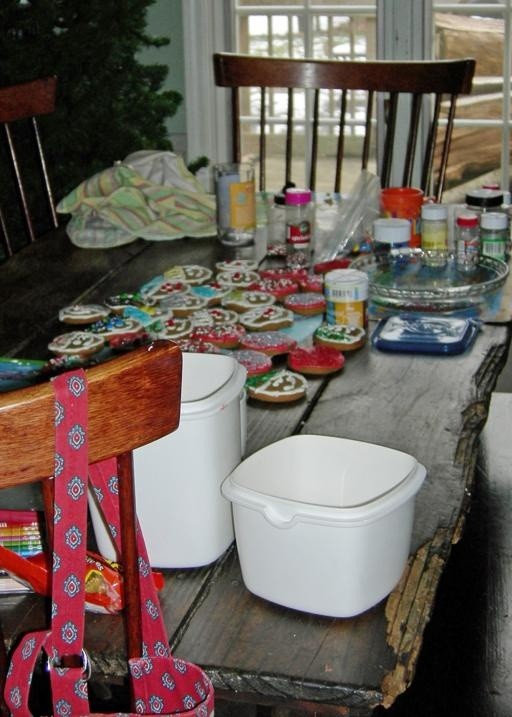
[3,368,218,717]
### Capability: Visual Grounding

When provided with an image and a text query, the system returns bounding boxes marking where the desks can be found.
[0,192,512,717]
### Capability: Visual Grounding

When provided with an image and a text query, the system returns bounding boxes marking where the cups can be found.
[209,160,257,249]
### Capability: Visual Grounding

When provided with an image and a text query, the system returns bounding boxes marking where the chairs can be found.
[0,340,183,717]
[0,73,59,257]
[211,51,476,205]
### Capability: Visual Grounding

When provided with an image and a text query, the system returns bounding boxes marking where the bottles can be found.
[321,266,371,338]
[371,187,511,261]
[264,185,315,256]
